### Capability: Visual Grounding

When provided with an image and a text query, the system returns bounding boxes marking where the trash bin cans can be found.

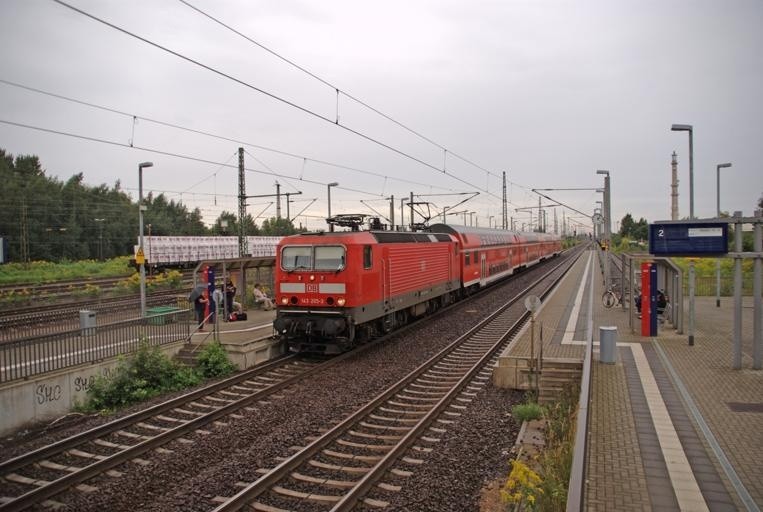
[79,310,96,337]
[599,327,617,365]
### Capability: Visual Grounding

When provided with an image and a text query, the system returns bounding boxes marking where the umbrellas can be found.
[189,284,206,303]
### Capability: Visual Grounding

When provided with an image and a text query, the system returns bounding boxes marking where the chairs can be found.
[656,302,673,332]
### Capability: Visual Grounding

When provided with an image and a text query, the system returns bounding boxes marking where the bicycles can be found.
[602,283,630,311]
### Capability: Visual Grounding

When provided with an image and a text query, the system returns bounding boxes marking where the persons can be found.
[657,290,666,314]
[253,284,276,311]
[222,278,236,312]
[195,295,208,330]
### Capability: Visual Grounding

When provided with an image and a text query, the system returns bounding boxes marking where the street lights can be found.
[671,124,695,347]
[716,163,732,307]
[595,169,611,306]
[138,161,154,326]
[327,182,338,233]
[443,206,449,224]
[401,197,409,232]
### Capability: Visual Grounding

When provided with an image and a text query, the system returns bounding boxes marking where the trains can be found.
[272,213,562,355]
